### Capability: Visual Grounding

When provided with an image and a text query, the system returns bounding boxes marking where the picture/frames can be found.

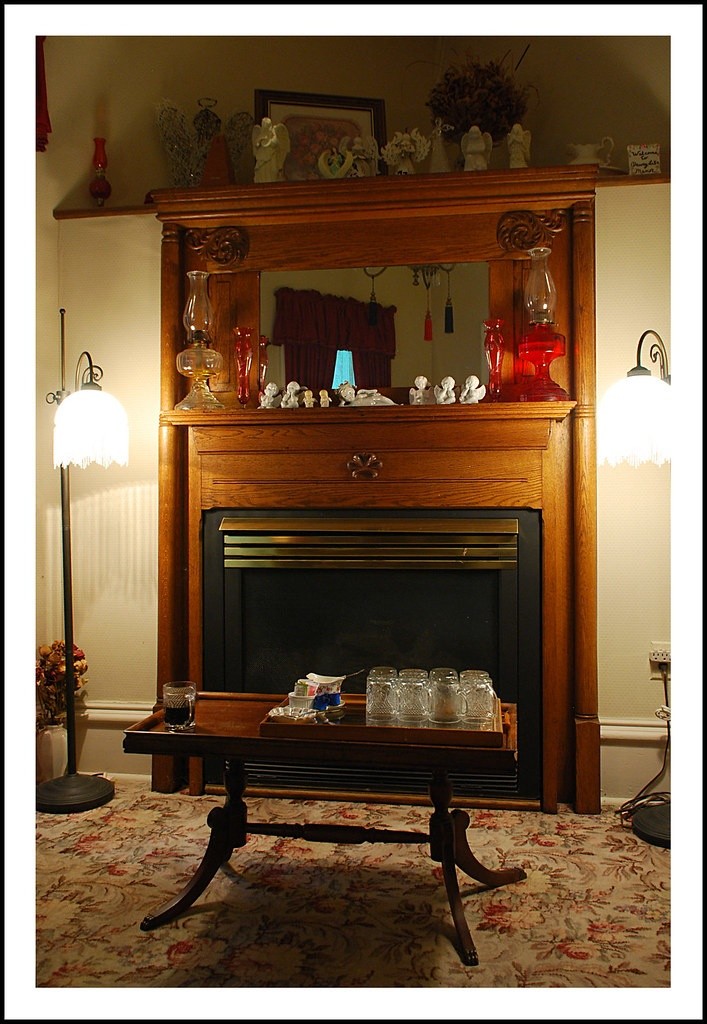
[253,88,389,176]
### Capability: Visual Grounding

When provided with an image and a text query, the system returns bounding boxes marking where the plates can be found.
[329,700,346,711]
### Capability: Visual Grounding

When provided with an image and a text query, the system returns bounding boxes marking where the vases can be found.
[452,142,465,172]
[36,723,68,779]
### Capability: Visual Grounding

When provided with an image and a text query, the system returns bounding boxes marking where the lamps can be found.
[90,139,112,211]
[36,309,129,815]
[173,271,226,410]
[516,247,571,402]
[595,330,671,849]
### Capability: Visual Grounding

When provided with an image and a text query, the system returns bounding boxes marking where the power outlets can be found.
[649,640,671,680]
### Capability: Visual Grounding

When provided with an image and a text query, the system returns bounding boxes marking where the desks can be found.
[122,689,527,967]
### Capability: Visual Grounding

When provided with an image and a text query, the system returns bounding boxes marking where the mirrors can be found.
[259,261,492,389]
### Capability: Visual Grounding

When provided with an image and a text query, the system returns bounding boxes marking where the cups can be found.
[460,670,489,679]
[288,692,316,710]
[400,669,428,680]
[366,676,396,721]
[396,678,428,722]
[369,667,396,679]
[428,678,468,723]
[429,668,458,680]
[163,681,197,732]
[460,677,499,725]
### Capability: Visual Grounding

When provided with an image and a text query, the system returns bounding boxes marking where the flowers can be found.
[36,639,89,733]
[405,47,542,148]
